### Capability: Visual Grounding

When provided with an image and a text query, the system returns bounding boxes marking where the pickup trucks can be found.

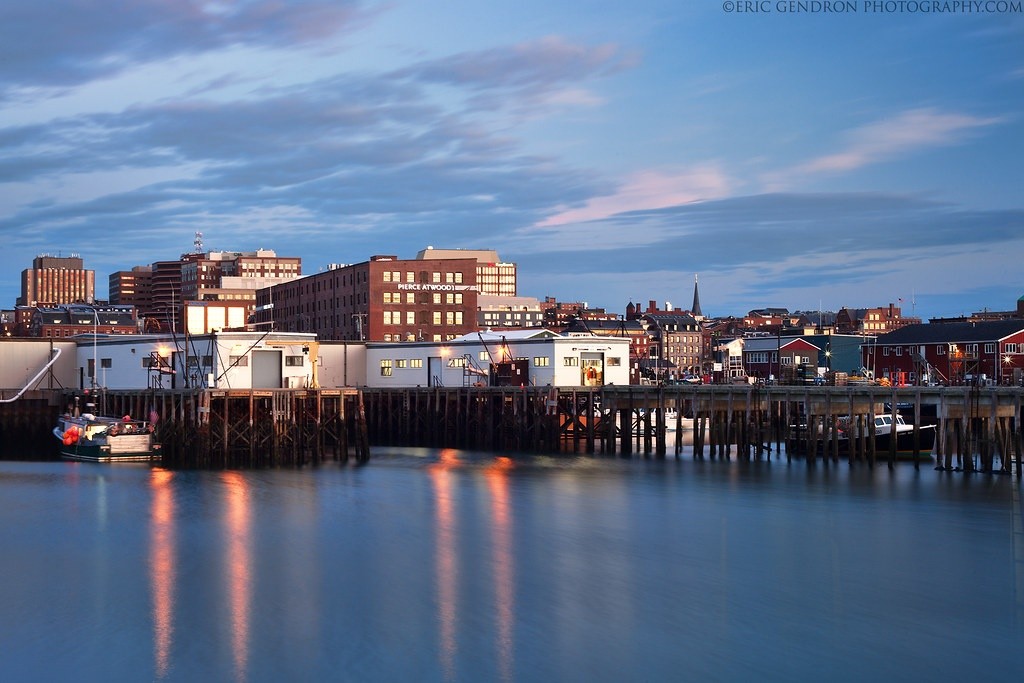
[680,374,705,384]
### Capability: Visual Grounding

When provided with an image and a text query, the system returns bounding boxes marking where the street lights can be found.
[825,352,830,379]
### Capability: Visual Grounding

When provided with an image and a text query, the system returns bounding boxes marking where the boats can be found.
[784,413,938,456]
[52,375,164,463]
